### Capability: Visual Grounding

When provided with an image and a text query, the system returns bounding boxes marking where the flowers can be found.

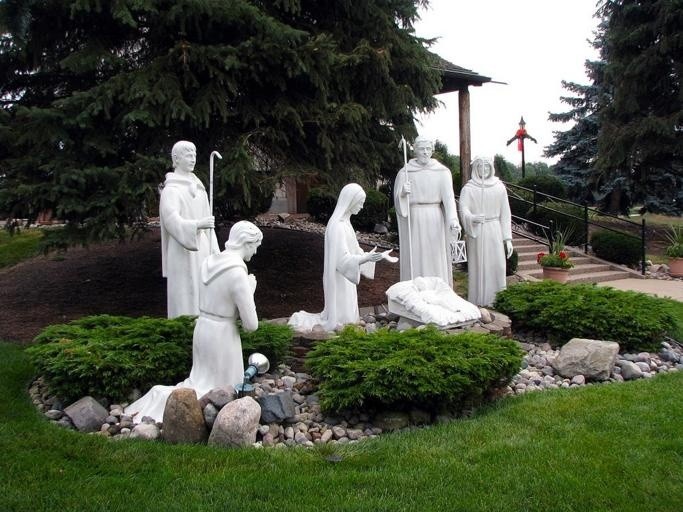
[535,219,578,270]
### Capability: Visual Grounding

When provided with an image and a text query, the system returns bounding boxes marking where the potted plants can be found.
[662,222,683,278]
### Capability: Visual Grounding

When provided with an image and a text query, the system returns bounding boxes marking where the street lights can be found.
[518,115,527,179]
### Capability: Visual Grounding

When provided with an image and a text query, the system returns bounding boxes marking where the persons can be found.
[158,139,221,321]
[458,155,513,308]
[288,181,401,335]
[393,134,461,294]
[123,217,265,427]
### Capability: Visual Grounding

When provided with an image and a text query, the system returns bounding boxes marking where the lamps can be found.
[240,352,271,398]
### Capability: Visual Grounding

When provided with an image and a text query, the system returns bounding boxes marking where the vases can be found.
[541,266,569,286]
